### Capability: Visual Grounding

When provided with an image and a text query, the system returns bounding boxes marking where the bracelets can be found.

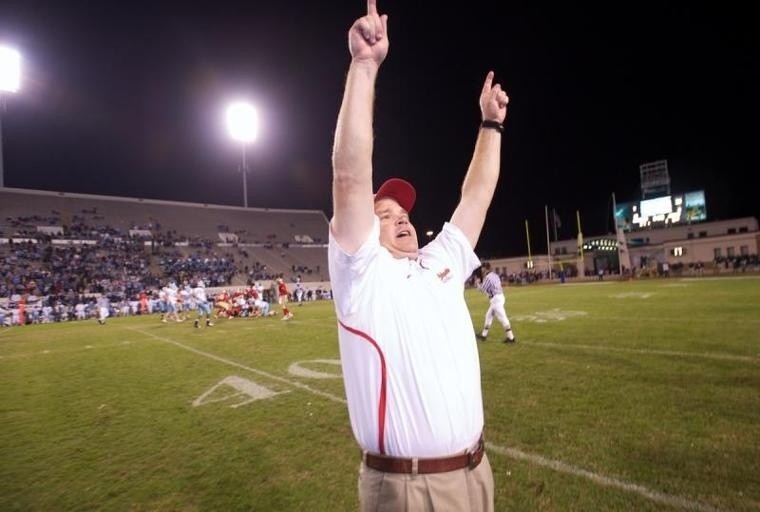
[479,115,507,134]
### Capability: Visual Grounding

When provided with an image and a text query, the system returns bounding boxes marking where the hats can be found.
[372,177,416,213]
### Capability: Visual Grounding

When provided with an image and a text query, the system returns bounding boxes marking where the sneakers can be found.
[503,337,515,343]
[161,312,294,329]
[97,320,106,325]
[475,333,487,341]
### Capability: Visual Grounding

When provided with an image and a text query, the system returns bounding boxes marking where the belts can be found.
[359,433,485,474]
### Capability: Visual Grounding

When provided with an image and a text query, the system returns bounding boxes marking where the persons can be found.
[326,0,510,511]
[466,253,760,284]
[473,259,516,344]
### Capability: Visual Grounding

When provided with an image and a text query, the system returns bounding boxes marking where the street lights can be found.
[0,46,21,93]
[226,100,258,208]
